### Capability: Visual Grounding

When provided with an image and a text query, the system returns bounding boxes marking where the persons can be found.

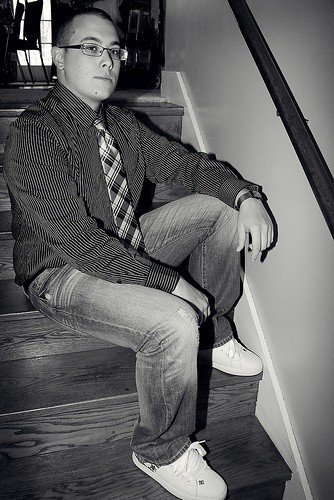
[2,7,274,500]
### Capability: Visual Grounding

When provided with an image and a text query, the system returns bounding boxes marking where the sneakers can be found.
[197,338,262,376]
[132,440,227,500]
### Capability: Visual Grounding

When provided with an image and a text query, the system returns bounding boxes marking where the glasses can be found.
[58,43,128,60]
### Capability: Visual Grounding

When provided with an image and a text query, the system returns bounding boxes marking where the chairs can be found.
[0,0,50,84]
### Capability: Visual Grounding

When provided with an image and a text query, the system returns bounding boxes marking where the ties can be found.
[93,117,149,262]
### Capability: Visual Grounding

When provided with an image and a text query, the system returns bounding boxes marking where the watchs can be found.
[237,190,263,209]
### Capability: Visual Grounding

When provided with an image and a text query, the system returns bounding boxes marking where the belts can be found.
[23,266,46,293]
